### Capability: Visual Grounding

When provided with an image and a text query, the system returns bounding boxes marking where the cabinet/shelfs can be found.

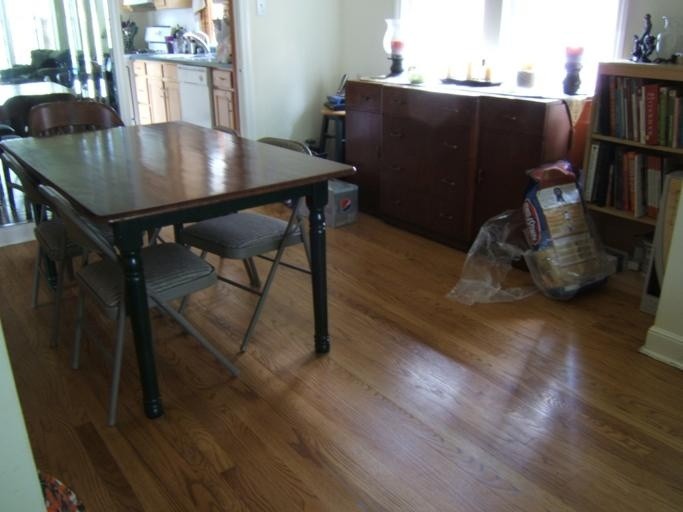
[161,61,184,122]
[131,57,165,124]
[211,67,235,131]
[581,57,683,301]
[379,86,477,254]
[342,79,379,216]
[153,0,201,11]
[474,96,586,272]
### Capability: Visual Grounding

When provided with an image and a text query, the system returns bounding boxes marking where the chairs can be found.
[178,133,316,358]
[0,147,140,343]
[35,182,240,428]
[0,48,74,87]
[25,100,139,289]
[0,95,78,220]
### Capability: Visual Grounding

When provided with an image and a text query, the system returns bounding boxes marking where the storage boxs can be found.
[297,178,357,230]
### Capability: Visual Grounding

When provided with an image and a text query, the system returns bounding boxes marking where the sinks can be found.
[152,52,214,60]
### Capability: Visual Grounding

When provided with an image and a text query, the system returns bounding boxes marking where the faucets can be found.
[183,30,211,55]
[481,56,493,81]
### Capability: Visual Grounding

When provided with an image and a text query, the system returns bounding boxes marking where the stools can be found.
[317,108,347,163]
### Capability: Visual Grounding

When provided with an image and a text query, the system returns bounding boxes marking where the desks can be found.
[0,120,357,419]
[0,81,76,107]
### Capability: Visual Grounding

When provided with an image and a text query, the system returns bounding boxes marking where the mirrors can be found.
[0,1,133,248]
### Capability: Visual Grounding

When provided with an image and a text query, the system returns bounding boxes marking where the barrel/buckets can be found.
[320,112,344,160]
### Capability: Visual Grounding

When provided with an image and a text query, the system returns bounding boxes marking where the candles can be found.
[565,46,585,57]
[390,39,401,50]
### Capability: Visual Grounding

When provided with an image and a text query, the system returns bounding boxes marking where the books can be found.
[583,75,683,219]
